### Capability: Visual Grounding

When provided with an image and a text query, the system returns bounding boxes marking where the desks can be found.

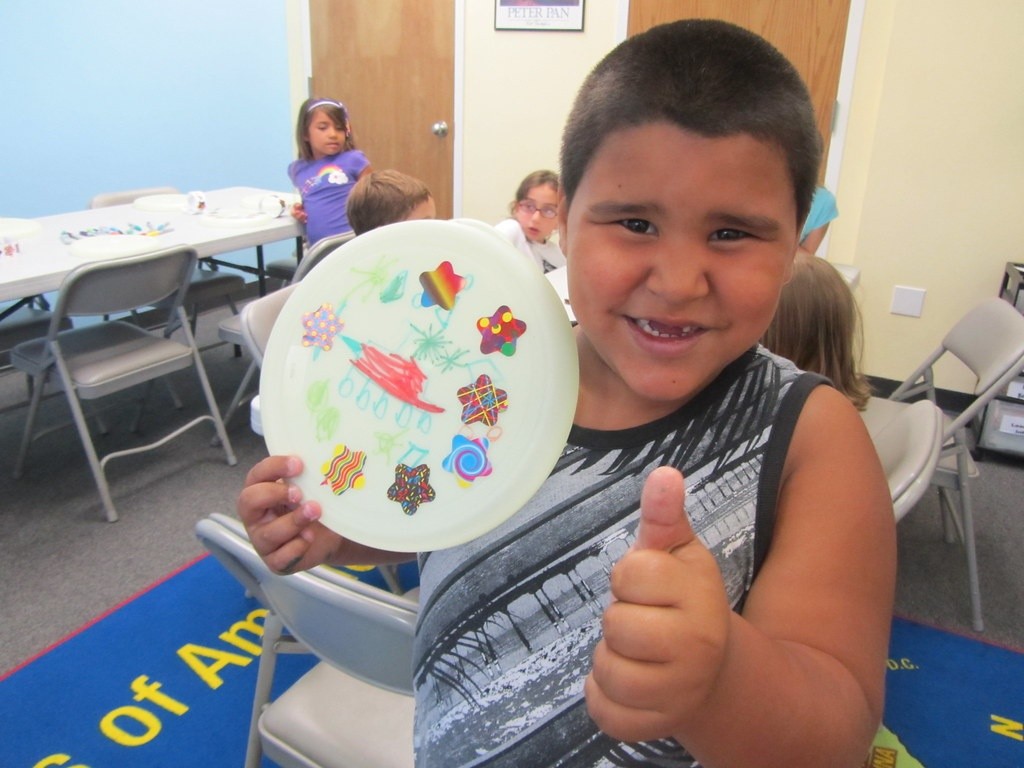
[0,187,306,357]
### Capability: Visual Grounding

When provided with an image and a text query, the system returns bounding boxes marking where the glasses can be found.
[518,198,560,221]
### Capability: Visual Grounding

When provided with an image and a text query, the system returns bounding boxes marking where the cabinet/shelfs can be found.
[965,262,1024,460]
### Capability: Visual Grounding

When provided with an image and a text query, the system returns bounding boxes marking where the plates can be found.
[260,214,579,552]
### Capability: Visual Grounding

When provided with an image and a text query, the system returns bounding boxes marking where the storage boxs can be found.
[978,376,1024,456]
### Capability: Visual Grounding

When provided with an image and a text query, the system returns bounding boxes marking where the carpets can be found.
[0,552,1024,768]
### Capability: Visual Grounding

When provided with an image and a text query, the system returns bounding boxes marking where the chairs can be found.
[856,297,1024,631]
[0,187,399,597]
[196,512,420,768]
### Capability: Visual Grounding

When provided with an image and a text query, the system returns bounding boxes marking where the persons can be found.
[287,97,373,247]
[493,170,567,274]
[795,186,837,260]
[237,20,899,768]
[757,256,873,413]
[345,170,435,237]
